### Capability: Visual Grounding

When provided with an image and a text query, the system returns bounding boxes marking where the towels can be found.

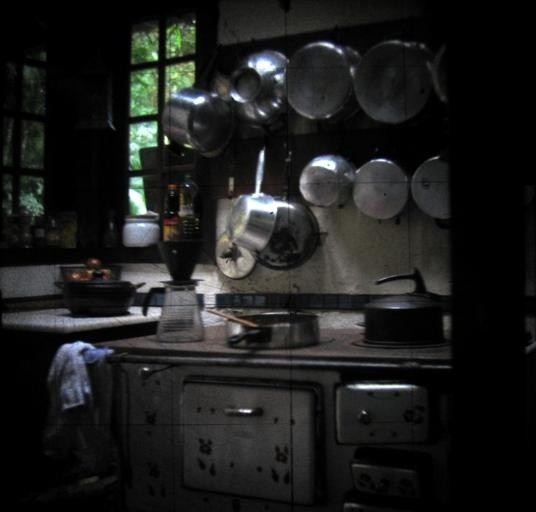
[44,339,98,412]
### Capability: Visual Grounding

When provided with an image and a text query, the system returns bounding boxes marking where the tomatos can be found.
[70,258,111,282]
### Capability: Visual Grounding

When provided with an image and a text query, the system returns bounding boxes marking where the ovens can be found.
[180,378,428,512]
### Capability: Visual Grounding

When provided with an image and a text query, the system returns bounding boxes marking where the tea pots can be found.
[143,281,210,342]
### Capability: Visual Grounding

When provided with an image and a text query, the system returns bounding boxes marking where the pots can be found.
[364,266,448,348]
[161,41,449,281]
[226,309,320,346]
[53,270,147,316]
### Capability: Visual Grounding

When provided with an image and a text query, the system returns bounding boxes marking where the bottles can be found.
[0,212,119,251]
